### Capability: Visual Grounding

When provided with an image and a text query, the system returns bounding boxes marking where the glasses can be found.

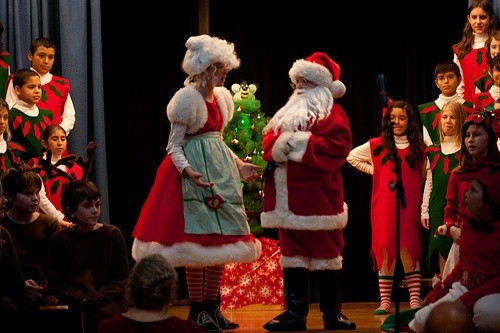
[294,79,311,85]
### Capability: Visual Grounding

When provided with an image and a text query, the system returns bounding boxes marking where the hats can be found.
[289,53,346,98]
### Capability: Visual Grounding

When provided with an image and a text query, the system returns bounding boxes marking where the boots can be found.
[188,303,221,331]
[310,256,356,329]
[205,300,238,329]
[263,255,311,331]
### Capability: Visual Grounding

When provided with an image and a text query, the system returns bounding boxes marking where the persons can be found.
[345,99,427,314]
[259,52,356,332]
[136,34,262,331]
[1,22,207,333]
[380,0,500,333]
